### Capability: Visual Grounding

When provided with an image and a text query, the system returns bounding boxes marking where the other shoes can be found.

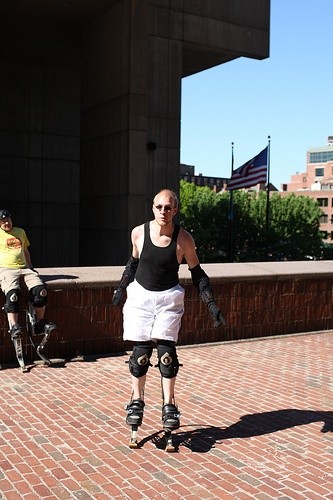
[33,318,56,337]
[7,324,24,341]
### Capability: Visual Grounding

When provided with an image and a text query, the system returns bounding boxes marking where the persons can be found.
[0,210,57,337]
[112,190,226,429]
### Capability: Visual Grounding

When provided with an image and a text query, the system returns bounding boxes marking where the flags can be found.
[225,146,267,190]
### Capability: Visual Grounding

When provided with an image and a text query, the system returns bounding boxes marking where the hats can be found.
[0,210,13,220]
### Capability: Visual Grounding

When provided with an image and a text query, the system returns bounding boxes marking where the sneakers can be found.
[125,398,146,426]
[161,402,180,429]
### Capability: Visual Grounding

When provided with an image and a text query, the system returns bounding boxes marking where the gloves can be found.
[111,286,127,306]
[207,301,227,328]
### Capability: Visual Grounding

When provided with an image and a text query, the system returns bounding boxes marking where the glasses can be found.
[154,204,172,211]
